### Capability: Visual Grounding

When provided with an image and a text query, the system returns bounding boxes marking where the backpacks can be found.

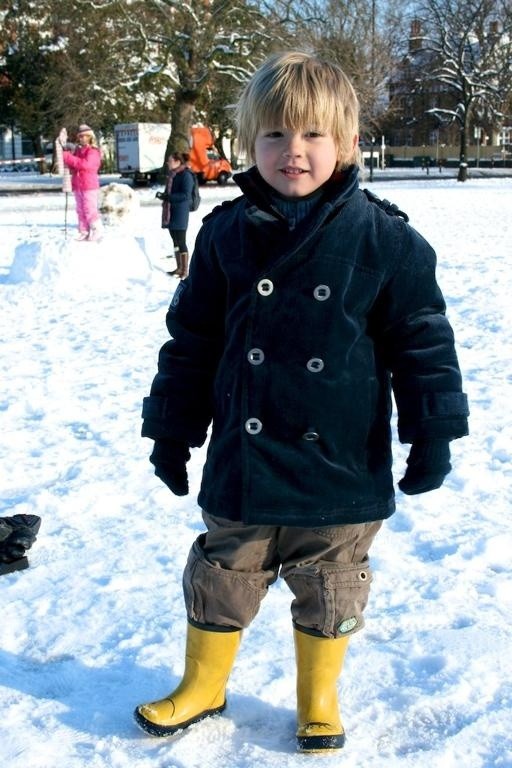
[189,170,202,213]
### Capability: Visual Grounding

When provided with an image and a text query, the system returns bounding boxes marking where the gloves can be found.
[149,435,196,497]
[398,433,454,501]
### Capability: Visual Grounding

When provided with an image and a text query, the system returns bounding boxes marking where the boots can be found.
[290,617,356,754]
[166,251,190,280]
[131,615,247,741]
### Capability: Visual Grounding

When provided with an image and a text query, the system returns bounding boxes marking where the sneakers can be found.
[73,227,103,241]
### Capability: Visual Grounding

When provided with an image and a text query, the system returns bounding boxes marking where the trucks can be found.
[114,123,232,187]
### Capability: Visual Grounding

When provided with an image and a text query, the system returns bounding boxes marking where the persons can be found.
[61,122,103,242]
[132,50,472,755]
[156,152,195,278]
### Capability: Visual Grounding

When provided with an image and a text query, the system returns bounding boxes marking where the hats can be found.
[76,124,94,138]
